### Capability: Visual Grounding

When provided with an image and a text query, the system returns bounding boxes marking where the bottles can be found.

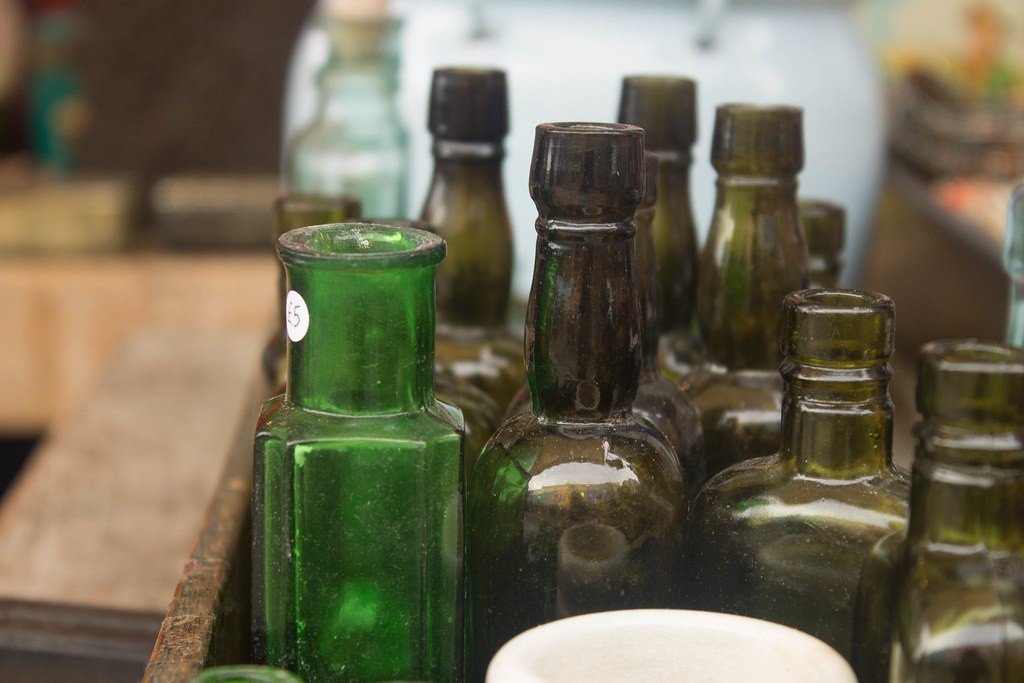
[287,17,408,213]
[616,76,712,386]
[690,291,911,683]
[460,122,685,683]
[268,199,364,399]
[424,67,527,452]
[851,340,1024,683]
[802,202,845,286]
[249,223,464,683]
[661,101,803,455]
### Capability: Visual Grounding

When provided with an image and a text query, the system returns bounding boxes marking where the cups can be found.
[485,609,856,683]
